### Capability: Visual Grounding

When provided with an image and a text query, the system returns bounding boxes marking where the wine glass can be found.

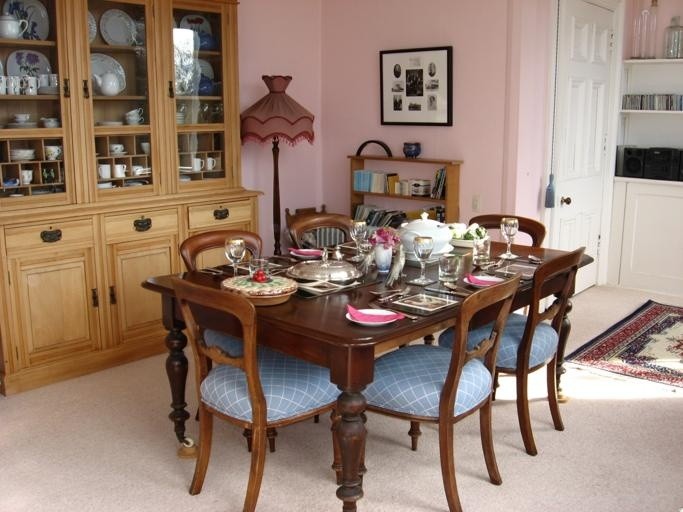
[224,238,246,275]
[349,220,367,261]
[414,217,519,284]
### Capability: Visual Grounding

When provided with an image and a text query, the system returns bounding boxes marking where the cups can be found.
[193,158,216,171]
[99,165,142,178]
[38,74,58,94]
[21,170,34,186]
[110,144,124,152]
[249,258,265,274]
[44,146,61,160]
[124,108,145,125]
[13,113,58,127]
[0,76,40,96]
[439,235,490,282]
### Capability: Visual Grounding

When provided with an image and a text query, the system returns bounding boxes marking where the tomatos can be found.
[253,270,267,282]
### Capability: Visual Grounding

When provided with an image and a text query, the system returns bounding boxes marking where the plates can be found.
[1,50,52,76]
[90,53,126,92]
[100,9,136,46]
[111,152,127,155]
[10,149,35,161]
[87,10,97,44]
[345,308,397,326]
[462,275,506,289]
[2,1,50,41]
[176,112,184,123]
[97,120,122,126]
[404,244,454,256]
[290,249,321,259]
[179,13,214,51]
[7,122,36,128]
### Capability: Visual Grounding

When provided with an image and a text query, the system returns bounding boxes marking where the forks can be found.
[369,285,412,301]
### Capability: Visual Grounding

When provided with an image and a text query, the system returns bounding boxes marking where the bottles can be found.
[631,0,659,59]
[664,17,683,58]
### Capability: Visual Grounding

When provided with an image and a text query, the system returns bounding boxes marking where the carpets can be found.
[564,299,683,389]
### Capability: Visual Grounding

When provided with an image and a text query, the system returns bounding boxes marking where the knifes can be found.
[423,287,468,298]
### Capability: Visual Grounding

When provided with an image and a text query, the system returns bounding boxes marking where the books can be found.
[430,167,447,200]
[622,93,683,111]
[354,204,406,230]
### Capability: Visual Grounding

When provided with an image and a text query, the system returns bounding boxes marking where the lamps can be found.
[241,75,314,256]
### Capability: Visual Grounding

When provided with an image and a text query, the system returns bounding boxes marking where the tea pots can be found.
[0,12,28,39]
[92,70,126,96]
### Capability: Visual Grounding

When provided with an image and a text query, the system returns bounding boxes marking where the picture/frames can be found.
[380,46,453,127]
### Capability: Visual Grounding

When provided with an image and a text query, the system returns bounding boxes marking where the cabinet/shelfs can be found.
[162,0,261,272]
[347,156,464,224]
[0,0,183,398]
[614,58,682,298]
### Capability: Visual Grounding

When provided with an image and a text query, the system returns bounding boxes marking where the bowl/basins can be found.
[398,212,451,252]
[140,143,150,153]
